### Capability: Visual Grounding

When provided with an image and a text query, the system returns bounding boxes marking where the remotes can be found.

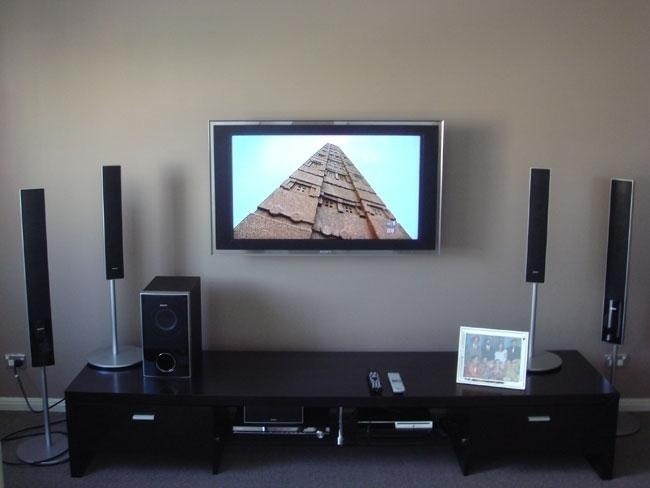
[387,372,406,394]
[367,370,383,392]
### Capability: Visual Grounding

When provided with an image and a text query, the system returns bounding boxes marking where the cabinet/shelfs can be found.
[64,350,621,480]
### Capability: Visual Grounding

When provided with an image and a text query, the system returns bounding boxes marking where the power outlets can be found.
[5,353,27,371]
[604,353,628,369]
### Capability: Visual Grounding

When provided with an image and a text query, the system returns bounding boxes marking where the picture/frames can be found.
[456,326,530,391]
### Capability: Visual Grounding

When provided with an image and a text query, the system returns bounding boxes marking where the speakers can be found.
[18,188,55,367]
[600,178,635,344]
[101,164,125,281]
[139,275,203,380]
[524,166,551,284]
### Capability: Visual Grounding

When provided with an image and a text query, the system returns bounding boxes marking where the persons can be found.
[464,335,520,383]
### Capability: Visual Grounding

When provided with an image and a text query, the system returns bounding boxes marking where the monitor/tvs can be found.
[207,118,446,257]
